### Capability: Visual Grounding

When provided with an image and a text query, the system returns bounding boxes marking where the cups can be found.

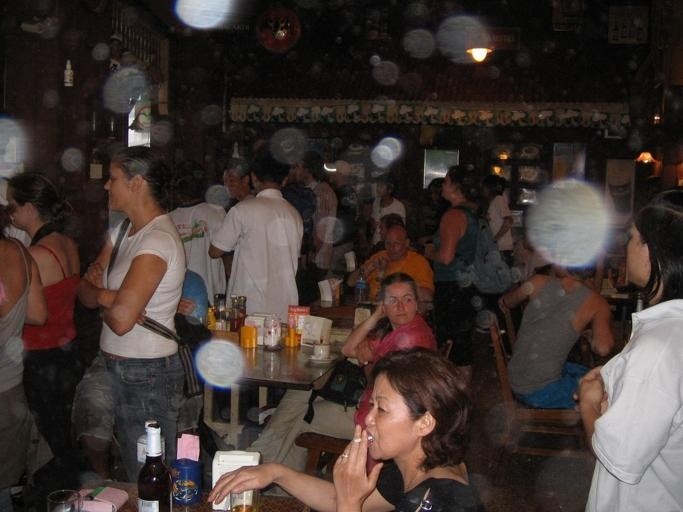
[229,486,261,512]
[171,461,203,505]
[313,342,330,360]
[46,489,84,512]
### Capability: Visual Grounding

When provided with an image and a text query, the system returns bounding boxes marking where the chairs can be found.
[295,304,632,512]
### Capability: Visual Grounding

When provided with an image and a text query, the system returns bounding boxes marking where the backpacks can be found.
[452,206,514,296]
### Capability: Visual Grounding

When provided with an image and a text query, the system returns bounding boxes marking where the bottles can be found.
[354,267,368,303]
[207,289,283,352]
[136,420,175,512]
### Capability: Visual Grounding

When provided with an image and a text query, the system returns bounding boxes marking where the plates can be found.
[302,353,340,364]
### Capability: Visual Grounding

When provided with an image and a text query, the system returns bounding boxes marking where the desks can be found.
[196,304,373,447]
[89,479,310,512]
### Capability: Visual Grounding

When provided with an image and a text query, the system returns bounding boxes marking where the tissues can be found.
[318,278,343,301]
[320,301,341,307]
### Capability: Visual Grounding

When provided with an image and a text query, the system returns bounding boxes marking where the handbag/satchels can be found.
[176,339,207,399]
[303,358,368,424]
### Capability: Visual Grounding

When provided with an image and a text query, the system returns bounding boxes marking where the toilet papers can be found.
[298,314,334,347]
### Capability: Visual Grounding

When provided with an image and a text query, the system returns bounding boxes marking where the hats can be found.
[322,159,353,177]
